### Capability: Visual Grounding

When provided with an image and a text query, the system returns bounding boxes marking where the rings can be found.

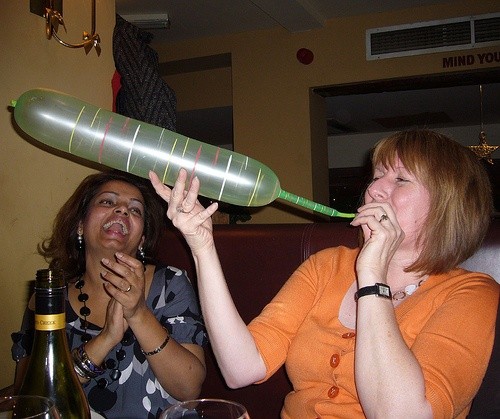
[379,214,388,223]
[177,206,187,213]
[124,285,132,293]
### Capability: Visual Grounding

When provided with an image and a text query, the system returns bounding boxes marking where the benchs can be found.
[140,223,499,418]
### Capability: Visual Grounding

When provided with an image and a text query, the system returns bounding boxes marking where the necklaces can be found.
[391,279,425,301]
[74,265,147,388]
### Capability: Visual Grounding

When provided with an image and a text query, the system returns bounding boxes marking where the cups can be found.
[159,398,250,419]
[0,395,63,419]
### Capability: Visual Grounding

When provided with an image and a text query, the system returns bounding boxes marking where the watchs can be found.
[355,283,392,302]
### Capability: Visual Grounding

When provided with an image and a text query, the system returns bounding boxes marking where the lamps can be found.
[120,14,169,30]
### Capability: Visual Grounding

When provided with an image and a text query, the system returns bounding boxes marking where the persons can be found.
[149,129,500,419]
[11,168,209,419]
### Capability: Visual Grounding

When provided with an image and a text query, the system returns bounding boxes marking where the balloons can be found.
[10,87,362,218]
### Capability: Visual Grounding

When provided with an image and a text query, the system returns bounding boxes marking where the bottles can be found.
[12,269,92,419]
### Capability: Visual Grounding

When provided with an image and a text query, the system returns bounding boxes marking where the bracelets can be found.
[77,344,103,374]
[71,352,96,379]
[74,349,94,373]
[71,359,86,378]
[141,331,170,355]
